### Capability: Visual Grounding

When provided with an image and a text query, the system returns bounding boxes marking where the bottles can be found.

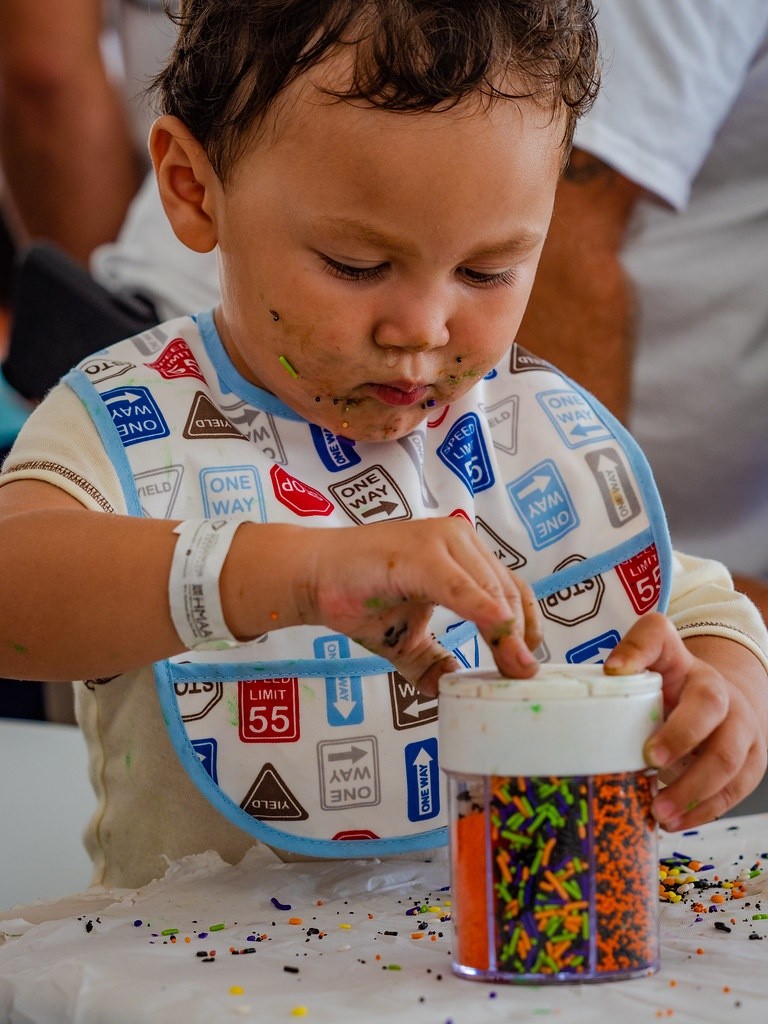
[437,663,666,987]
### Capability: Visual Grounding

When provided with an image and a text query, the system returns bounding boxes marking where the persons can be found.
[0,0,768,890]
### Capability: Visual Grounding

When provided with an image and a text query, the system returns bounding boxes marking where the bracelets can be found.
[168,512,265,649]
